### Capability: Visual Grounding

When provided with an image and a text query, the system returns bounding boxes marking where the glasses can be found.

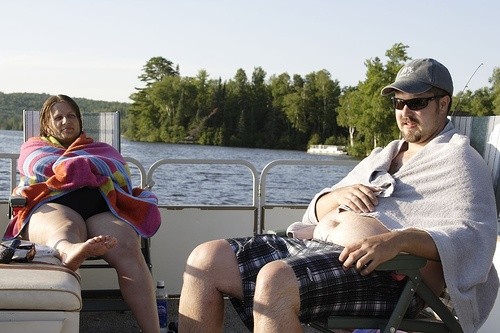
[394,94,449,111]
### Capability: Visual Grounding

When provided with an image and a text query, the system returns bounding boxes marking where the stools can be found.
[0,239,83,333]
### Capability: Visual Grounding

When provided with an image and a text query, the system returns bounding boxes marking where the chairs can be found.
[266,115,500,333]
[7,109,153,315]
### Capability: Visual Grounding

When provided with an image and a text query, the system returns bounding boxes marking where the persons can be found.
[12,95,159,333]
[178,58,498,333]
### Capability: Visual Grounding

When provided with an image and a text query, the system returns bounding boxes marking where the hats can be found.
[381,58,454,97]
